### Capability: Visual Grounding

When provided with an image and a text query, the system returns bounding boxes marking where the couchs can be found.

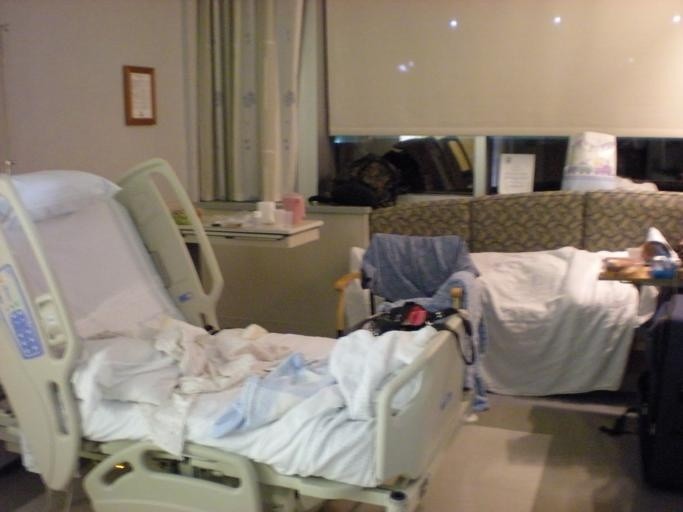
[333,183,682,418]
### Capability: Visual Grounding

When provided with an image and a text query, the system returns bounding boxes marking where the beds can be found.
[1,158,480,511]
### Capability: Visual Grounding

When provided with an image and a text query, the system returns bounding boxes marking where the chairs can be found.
[335,231,486,414]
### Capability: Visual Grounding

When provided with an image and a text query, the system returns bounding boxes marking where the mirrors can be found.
[320,133,486,199]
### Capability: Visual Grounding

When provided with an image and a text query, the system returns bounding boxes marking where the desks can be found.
[166,207,320,287]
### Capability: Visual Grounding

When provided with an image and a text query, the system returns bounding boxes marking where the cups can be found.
[276,211,292,228]
[283,195,303,223]
[257,202,274,223]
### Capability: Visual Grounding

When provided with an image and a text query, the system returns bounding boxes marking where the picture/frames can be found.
[121,63,157,127]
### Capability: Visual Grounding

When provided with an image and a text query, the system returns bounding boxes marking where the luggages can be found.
[638,291,683,493]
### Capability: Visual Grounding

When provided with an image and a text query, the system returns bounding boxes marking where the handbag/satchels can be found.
[308,148,427,209]
[370,302,433,336]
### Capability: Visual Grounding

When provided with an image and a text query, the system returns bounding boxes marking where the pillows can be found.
[0,168,125,231]
